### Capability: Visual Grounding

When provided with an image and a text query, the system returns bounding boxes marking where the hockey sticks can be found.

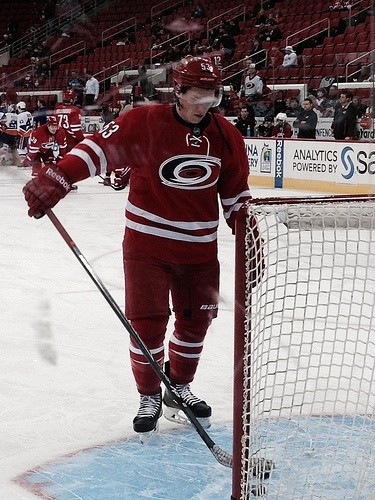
[44,205,276,478]
[71,185,78,191]
[97,174,124,191]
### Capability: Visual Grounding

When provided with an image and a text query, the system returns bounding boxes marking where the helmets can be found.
[275,113,288,123]
[16,101,26,108]
[173,55,220,89]
[46,117,58,127]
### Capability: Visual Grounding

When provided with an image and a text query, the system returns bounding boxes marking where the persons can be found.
[235,105,257,137]
[23,56,265,440]
[307,64,375,119]
[99,65,159,185]
[66,69,86,108]
[329,0,357,11]
[22,40,53,88]
[293,98,317,139]
[124,30,136,45]
[0,97,50,167]
[149,3,242,65]
[23,118,78,193]
[271,113,292,138]
[54,89,86,145]
[330,90,359,140]
[0,0,80,55]
[248,0,282,70]
[257,115,275,138]
[209,45,304,118]
[84,69,99,106]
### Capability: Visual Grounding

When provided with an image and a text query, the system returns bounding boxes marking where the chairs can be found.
[0,0,374,143]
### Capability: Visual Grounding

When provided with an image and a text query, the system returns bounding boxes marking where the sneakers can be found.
[133,390,163,443]
[163,361,212,428]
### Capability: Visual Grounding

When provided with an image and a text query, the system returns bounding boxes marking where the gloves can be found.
[22,155,31,167]
[15,135,21,141]
[104,177,111,186]
[248,216,264,288]
[20,163,78,219]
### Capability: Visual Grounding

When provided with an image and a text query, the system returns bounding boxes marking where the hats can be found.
[250,64,258,70]
[285,46,292,51]
